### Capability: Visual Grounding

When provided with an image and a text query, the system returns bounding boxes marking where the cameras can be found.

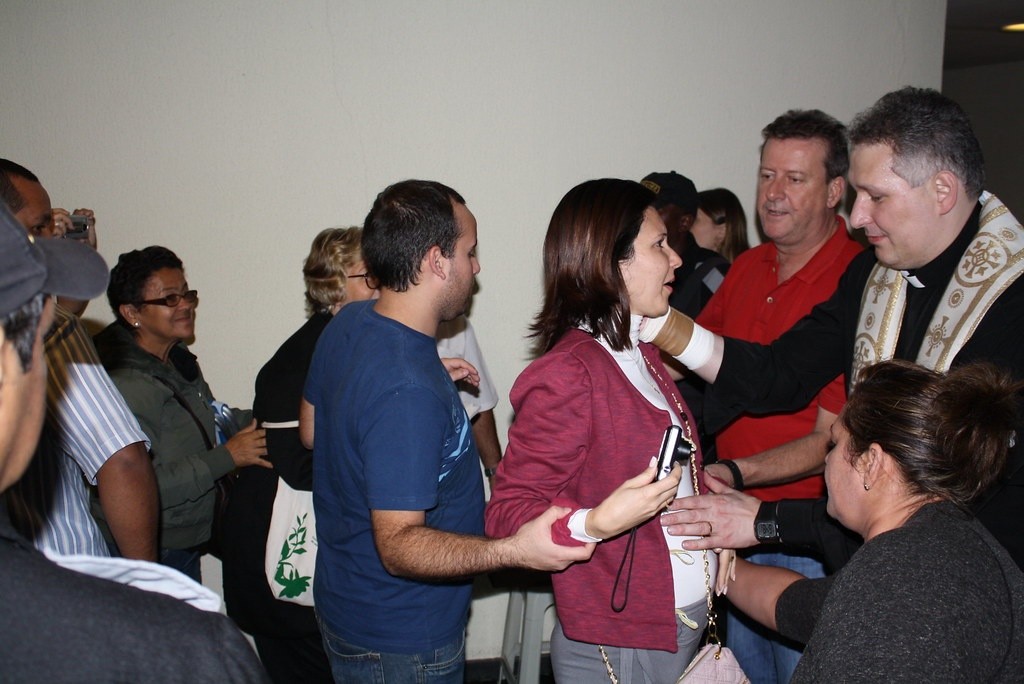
[654,425,692,482]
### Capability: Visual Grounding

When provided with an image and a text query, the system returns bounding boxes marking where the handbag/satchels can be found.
[266,476,318,606]
[677,644,751,684]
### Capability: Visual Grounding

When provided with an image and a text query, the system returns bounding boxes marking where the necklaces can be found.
[626,350,660,394]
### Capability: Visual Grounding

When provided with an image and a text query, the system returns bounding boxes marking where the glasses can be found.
[128,290,198,307]
[347,269,378,290]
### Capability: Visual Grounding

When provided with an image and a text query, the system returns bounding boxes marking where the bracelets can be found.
[715,459,743,491]
[485,468,496,476]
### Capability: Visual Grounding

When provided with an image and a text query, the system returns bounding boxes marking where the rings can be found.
[731,557,733,561]
[707,522,712,536]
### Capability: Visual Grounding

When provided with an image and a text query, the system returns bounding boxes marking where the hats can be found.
[641,170,699,216]
[0,203,110,314]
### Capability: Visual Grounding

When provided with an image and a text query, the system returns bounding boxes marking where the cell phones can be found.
[62,214,89,239]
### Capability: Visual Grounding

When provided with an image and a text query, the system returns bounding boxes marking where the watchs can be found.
[755,501,779,544]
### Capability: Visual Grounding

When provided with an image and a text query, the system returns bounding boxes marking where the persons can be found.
[660,85,1024,578]
[0,157,274,683]
[640,170,751,319]
[663,109,865,684]
[483,179,737,684]
[435,316,502,492]
[728,360,1024,684]
[221,226,479,684]
[300,179,597,684]
